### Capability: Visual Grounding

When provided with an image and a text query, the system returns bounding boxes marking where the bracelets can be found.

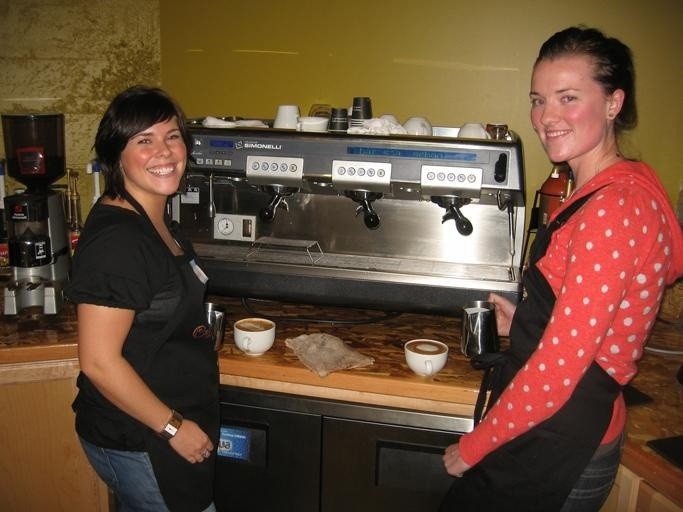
[155,412,183,440]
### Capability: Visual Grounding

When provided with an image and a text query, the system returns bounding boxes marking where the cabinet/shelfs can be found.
[0,358,112,511]
[213,385,477,512]
[597,462,683,512]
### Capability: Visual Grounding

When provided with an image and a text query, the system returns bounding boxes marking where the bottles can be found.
[68,194,83,261]
[0,170,13,281]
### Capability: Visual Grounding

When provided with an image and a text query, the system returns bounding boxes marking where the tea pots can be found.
[202,302,227,353]
[459,300,500,358]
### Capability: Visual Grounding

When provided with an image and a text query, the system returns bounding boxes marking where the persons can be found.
[72,84,221,507]
[443,28,683,511]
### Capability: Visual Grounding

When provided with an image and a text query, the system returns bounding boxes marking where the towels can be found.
[284,332,375,379]
[202,114,268,128]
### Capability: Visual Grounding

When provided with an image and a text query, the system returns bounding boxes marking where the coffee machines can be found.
[0,114,72,317]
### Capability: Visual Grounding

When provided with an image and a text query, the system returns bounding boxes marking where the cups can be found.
[295,116,329,133]
[404,338,449,378]
[272,105,300,129]
[457,122,492,140]
[487,123,508,141]
[233,318,277,356]
[379,114,398,125]
[328,107,349,132]
[402,117,433,137]
[350,96,372,126]
